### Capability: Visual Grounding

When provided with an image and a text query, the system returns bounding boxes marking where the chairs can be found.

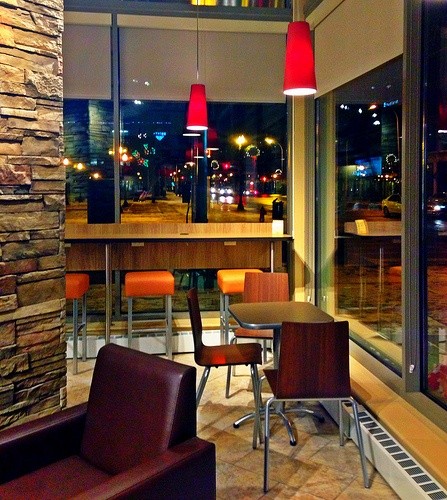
[255,319,370,493]
[224,271,291,399]
[184,286,267,447]
[0,343,217,500]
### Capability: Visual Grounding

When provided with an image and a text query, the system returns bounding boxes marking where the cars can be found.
[382,194,441,218]
[209,186,258,196]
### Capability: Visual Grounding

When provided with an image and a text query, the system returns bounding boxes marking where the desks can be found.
[342,219,402,332]
[226,301,335,446]
[62,221,293,345]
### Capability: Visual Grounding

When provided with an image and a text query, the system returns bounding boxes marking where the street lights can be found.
[169,165,195,197]
[265,137,283,187]
[369,104,401,177]
[63,157,100,206]
[235,135,246,212]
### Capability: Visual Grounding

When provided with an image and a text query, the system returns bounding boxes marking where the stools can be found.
[217,268,262,345]
[124,271,175,362]
[64,273,91,376]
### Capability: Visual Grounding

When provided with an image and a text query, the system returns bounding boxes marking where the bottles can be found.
[271,201,284,237]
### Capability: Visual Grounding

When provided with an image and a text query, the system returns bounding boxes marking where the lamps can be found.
[283,21,317,96]
[186,0,208,131]
[181,124,201,138]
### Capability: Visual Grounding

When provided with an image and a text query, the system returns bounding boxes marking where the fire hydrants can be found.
[259,206,266,223]
